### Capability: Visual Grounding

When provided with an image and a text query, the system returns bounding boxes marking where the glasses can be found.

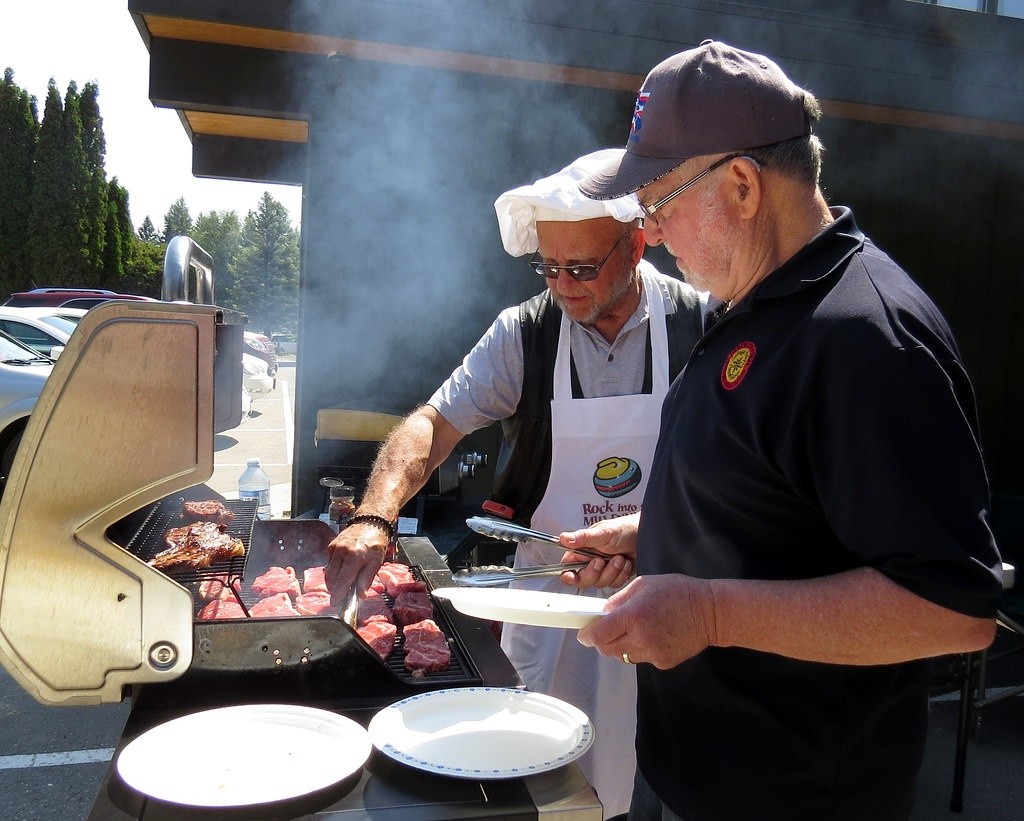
[528,220,645,281]
[638,154,762,226]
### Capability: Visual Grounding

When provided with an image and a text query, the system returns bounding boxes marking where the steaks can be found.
[147,499,245,572]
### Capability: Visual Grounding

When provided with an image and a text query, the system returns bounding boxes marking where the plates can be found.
[431,587,612,629]
[368,687,596,778]
[117,704,373,808]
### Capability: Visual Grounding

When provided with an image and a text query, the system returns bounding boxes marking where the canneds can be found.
[314,477,356,525]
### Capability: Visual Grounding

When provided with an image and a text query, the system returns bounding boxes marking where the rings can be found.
[622,652,634,665]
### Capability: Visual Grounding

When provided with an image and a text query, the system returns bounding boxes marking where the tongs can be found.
[337,580,359,629]
[452,516,636,587]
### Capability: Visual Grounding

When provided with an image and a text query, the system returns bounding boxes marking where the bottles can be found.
[329,486,356,536]
[239,458,271,521]
[314,477,344,519]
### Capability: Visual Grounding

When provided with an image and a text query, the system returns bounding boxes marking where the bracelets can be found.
[346,514,396,540]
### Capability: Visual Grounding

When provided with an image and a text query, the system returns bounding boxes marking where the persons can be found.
[558,39,1003,821]
[321,148,733,820]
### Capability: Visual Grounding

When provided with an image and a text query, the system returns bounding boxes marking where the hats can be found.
[577,39,813,201]
[494,149,646,257]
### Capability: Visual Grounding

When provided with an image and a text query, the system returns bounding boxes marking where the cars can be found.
[1,285,278,491]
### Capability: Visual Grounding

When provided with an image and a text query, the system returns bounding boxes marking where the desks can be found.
[292,494,490,578]
